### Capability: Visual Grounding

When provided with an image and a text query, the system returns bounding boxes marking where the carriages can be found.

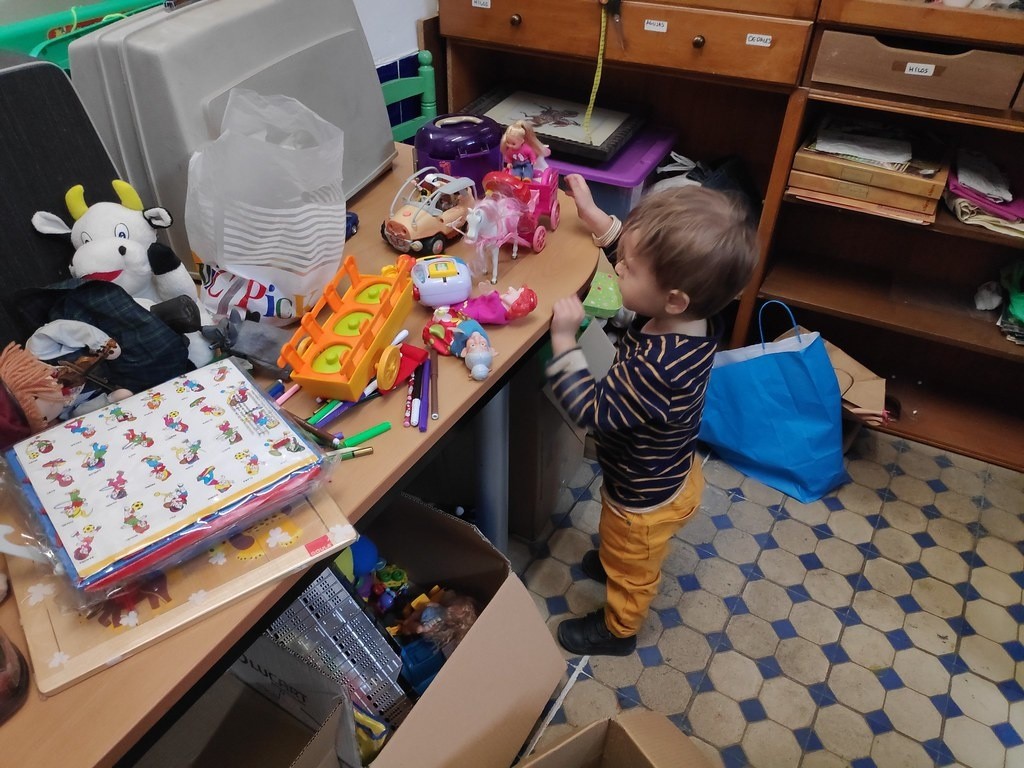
[452,120,561,285]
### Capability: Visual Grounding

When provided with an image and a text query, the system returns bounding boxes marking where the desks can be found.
[0,142,600,768]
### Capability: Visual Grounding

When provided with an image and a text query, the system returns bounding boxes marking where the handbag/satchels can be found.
[694,300,854,503]
[184,89,348,328]
[772,323,889,428]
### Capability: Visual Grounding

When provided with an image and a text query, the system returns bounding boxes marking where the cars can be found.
[345,211,360,243]
[380,166,478,256]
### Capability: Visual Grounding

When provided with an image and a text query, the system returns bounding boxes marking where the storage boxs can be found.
[506,318,621,547]
[543,125,684,224]
[127,490,716,768]
[788,121,954,217]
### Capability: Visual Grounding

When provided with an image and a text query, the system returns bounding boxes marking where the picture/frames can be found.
[454,77,655,162]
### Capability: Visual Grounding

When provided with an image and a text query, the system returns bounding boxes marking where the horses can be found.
[463,198,520,286]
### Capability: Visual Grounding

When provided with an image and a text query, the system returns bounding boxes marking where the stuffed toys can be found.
[31,179,216,368]
[0,318,132,451]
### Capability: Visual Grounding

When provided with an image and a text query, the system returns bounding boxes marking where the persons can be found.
[546,173,761,656]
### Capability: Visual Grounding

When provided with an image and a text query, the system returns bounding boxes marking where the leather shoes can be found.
[581,550,607,583]
[557,608,637,655]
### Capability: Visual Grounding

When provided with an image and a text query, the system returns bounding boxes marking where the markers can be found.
[261,347,440,460]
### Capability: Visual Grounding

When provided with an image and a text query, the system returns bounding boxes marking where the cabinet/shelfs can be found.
[438,1,1024,476]
